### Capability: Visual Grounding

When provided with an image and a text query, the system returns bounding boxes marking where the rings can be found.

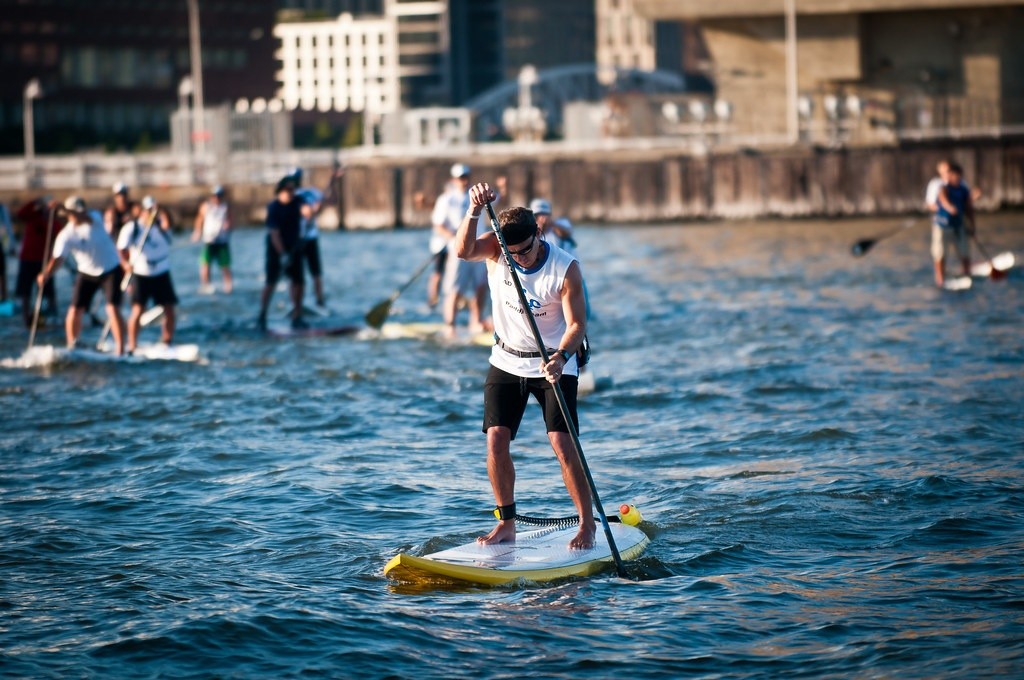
[556,372,558,376]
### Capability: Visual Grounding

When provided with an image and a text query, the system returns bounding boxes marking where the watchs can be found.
[556,350,571,362]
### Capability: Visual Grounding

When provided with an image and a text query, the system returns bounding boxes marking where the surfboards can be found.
[383,521,651,588]
[21,343,200,369]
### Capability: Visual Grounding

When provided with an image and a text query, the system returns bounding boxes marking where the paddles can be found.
[486,201,628,580]
[363,244,446,332]
[25,207,56,354]
[97,207,157,348]
[960,220,1008,281]
[850,213,932,257]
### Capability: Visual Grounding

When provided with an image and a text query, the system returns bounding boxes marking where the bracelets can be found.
[466,210,479,219]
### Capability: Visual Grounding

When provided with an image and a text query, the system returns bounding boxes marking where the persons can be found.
[0,200,63,315]
[925,159,974,286]
[415,161,507,336]
[530,198,578,258]
[104,181,142,235]
[454,183,597,550]
[257,167,344,329]
[192,185,236,291]
[117,194,179,352]
[37,195,125,355]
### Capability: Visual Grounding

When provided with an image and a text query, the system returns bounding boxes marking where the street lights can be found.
[23,79,41,191]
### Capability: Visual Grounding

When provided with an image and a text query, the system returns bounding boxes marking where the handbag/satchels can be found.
[576,334,592,368]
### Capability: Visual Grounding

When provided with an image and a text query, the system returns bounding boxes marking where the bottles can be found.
[619,505,641,526]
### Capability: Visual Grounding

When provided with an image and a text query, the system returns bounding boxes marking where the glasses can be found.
[507,232,537,256]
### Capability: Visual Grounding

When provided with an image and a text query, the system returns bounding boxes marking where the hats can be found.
[209,184,224,195]
[449,162,472,178]
[552,216,573,229]
[63,195,87,213]
[112,182,130,194]
[141,195,159,210]
[530,197,553,215]
